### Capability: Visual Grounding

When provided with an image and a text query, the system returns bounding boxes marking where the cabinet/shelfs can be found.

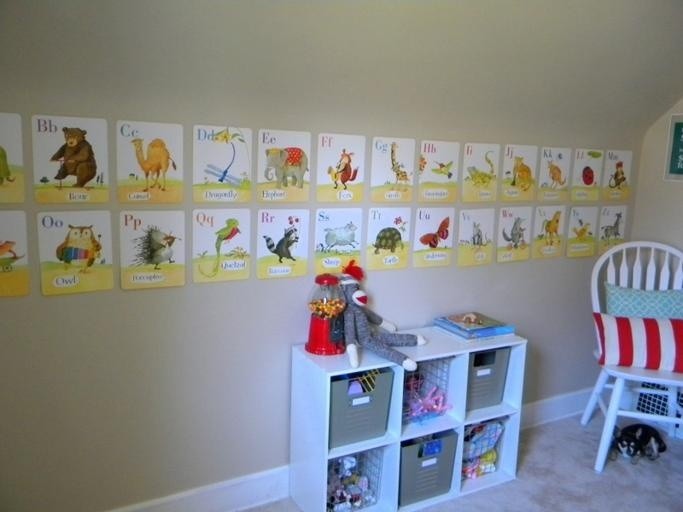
[288,322,530,512]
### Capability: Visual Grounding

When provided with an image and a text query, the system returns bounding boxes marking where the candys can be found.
[309,298,346,318]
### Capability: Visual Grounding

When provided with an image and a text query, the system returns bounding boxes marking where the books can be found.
[434,312,515,339]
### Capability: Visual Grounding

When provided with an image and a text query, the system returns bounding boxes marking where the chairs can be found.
[578,240,683,472]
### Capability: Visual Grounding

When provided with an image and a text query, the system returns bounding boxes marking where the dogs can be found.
[608,422,667,465]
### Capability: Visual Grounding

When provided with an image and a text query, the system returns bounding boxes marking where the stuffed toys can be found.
[340,261,427,371]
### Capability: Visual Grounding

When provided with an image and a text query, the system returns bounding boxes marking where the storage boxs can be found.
[465,346,511,412]
[328,366,394,449]
[398,429,458,508]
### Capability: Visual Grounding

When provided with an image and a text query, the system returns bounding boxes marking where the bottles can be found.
[304,273,347,356]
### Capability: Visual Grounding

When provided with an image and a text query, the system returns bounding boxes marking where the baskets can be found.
[404,356,455,424]
[327,450,381,511]
[462,415,511,481]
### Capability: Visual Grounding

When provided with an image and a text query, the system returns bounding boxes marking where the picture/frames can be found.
[661,114,682,182]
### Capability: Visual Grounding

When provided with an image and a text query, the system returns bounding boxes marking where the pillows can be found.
[590,311,683,372]
[601,280,683,320]
